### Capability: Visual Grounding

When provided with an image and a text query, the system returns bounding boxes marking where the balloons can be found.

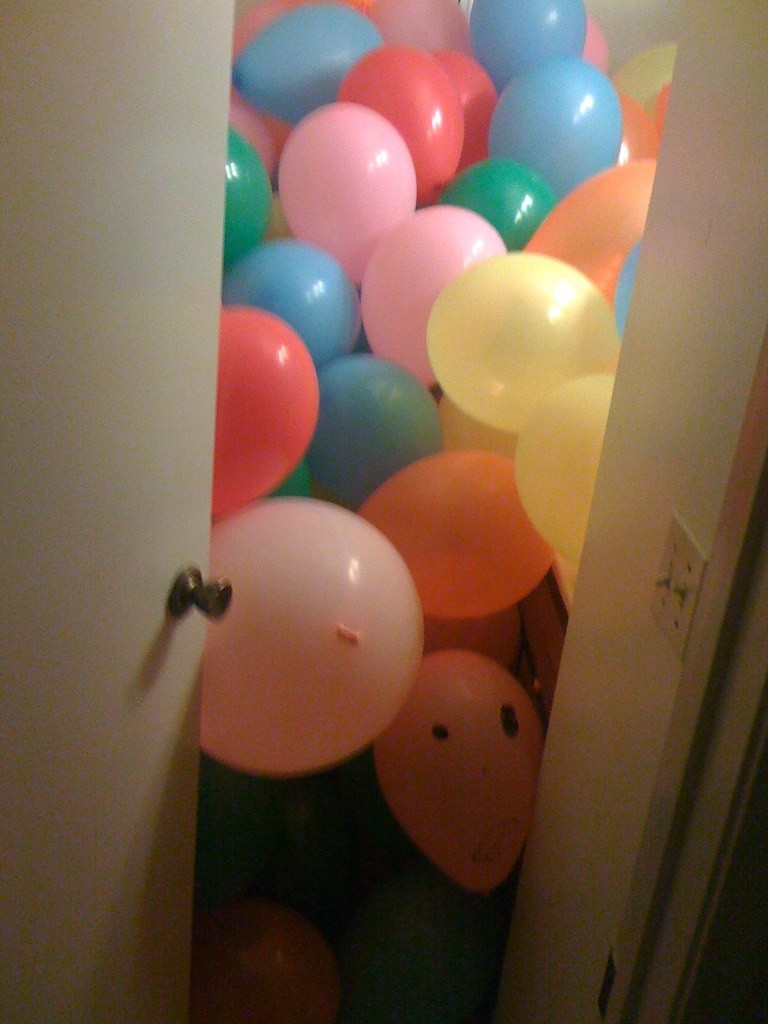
[189,0,674,1024]
